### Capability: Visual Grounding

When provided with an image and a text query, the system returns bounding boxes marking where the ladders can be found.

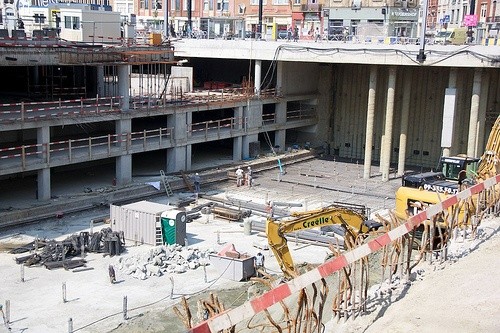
[154,216,163,246]
[160,169,174,197]
[180,169,195,193]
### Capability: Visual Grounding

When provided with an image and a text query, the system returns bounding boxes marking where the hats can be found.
[196,173,198,176]
[238,166,240,168]
[247,167,251,169]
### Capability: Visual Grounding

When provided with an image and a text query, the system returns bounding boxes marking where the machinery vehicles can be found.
[393,113,500,252]
[266,201,388,284]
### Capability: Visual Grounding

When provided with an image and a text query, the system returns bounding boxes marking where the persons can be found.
[193,173,201,193]
[18,18,26,37]
[255,253,264,277]
[323,141,328,155]
[120,23,475,43]
[235,166,252,188]
